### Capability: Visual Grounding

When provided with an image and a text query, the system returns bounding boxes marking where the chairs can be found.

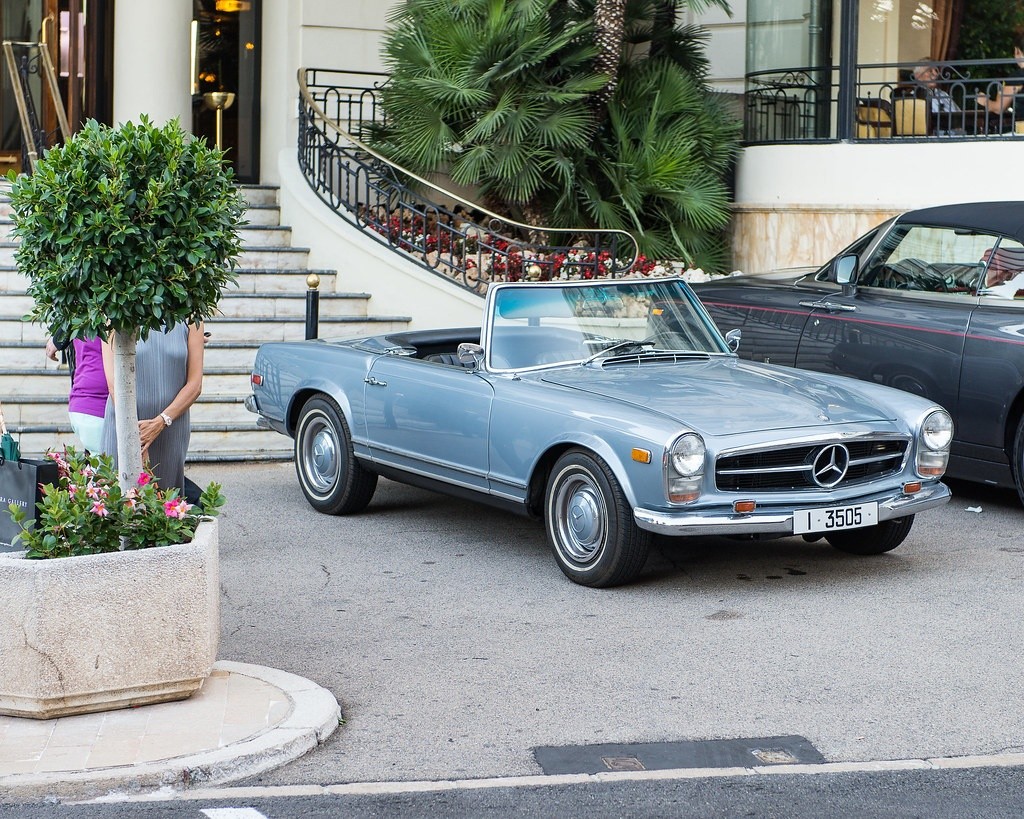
[424,352,512,369]
[888,85,932,136]
[536,349,613,366]
[856,97,892,138]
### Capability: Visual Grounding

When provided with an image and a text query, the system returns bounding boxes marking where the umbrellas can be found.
[0,410,21,462]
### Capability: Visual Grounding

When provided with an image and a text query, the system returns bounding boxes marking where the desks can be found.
[931,109,1000,133]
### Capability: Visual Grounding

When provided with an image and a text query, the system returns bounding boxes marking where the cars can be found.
[650,202,1024,506]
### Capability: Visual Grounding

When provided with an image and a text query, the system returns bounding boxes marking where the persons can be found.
[910,57,969,136]
[975,43,1024,133]
[45,311,209,498]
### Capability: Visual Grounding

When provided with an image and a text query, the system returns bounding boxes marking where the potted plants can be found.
[0,112,251,721]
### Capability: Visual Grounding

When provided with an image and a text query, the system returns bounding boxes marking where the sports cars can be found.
[246,275,961,592]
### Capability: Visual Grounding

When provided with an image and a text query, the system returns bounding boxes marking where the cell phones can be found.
[204,332,211,337]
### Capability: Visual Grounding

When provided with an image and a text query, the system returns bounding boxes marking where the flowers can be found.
[1,442,227,560]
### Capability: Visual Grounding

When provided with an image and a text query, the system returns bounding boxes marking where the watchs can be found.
[160,412,172,428]
